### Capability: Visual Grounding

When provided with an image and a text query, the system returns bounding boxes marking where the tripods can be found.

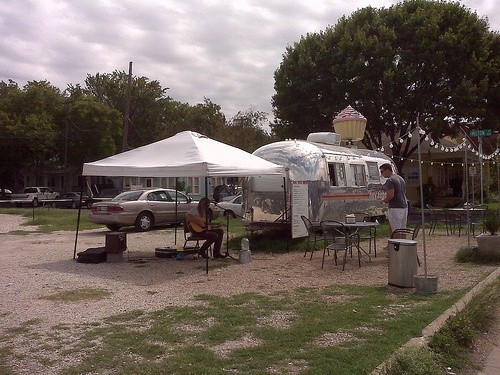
[211,204,246,260]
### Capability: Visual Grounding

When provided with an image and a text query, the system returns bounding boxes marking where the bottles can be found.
[241,237,249,251]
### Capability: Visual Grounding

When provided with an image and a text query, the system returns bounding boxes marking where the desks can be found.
[41,199,72,208]
[417,208,487,235]
[323,222,379,263]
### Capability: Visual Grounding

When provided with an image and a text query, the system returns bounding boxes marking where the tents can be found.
[73,131,289,274]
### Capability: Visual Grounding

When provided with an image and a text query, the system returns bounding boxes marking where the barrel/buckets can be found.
[388,238,417,288]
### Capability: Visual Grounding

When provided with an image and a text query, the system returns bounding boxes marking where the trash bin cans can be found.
[388,238,417,287]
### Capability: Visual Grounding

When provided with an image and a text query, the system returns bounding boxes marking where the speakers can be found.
[106,232,128,253]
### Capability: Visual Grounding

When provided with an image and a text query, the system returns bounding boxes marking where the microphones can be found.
[211,201,219,204]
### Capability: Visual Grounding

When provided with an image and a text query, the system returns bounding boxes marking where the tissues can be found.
[345,214,356,224]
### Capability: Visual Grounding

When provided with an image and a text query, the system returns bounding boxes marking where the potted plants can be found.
[177,181,187,195]
[475,214,500,256]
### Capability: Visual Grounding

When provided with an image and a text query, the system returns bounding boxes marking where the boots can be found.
[213,243,227,259]
[197,239,213,259]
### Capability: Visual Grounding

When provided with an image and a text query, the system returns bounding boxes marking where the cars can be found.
[89,188,220,232]
[11,186,60,208]
[51,192,92,208]
[216,191,243,221]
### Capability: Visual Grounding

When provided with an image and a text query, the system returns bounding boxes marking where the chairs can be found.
[182,218,213,258]
[301,215,422,272]
[426,204,488,239]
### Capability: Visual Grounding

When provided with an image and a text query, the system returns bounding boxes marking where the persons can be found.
[186,198,227,259]
[379,163,408,233]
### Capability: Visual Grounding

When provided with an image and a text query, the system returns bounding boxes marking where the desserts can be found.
[333,105,367,142]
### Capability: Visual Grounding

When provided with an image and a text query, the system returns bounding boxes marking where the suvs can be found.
[213,185,237,201]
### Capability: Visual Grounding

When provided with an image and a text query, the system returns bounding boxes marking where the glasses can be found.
[381,169,385,173]
